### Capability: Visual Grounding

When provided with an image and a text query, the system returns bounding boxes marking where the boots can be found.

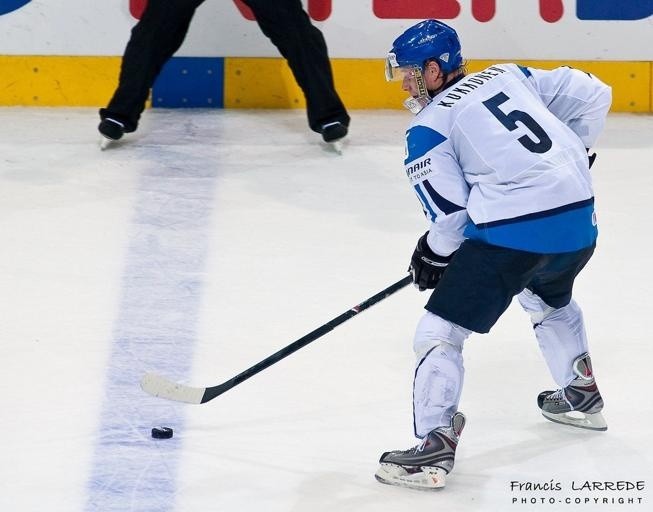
[378,412,465,474]
[99,117,125,140]
[537,354,603,415]
[321,121,347,142]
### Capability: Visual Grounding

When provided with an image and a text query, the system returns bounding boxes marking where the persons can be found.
[374,18,615,476]
[95,0,350,144]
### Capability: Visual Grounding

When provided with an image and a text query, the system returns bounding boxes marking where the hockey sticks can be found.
[139,275,414,403]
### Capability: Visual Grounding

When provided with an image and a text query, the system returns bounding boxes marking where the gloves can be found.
[408,231,454,292]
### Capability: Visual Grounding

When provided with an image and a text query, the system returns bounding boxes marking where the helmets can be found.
[383,20,463,81]
